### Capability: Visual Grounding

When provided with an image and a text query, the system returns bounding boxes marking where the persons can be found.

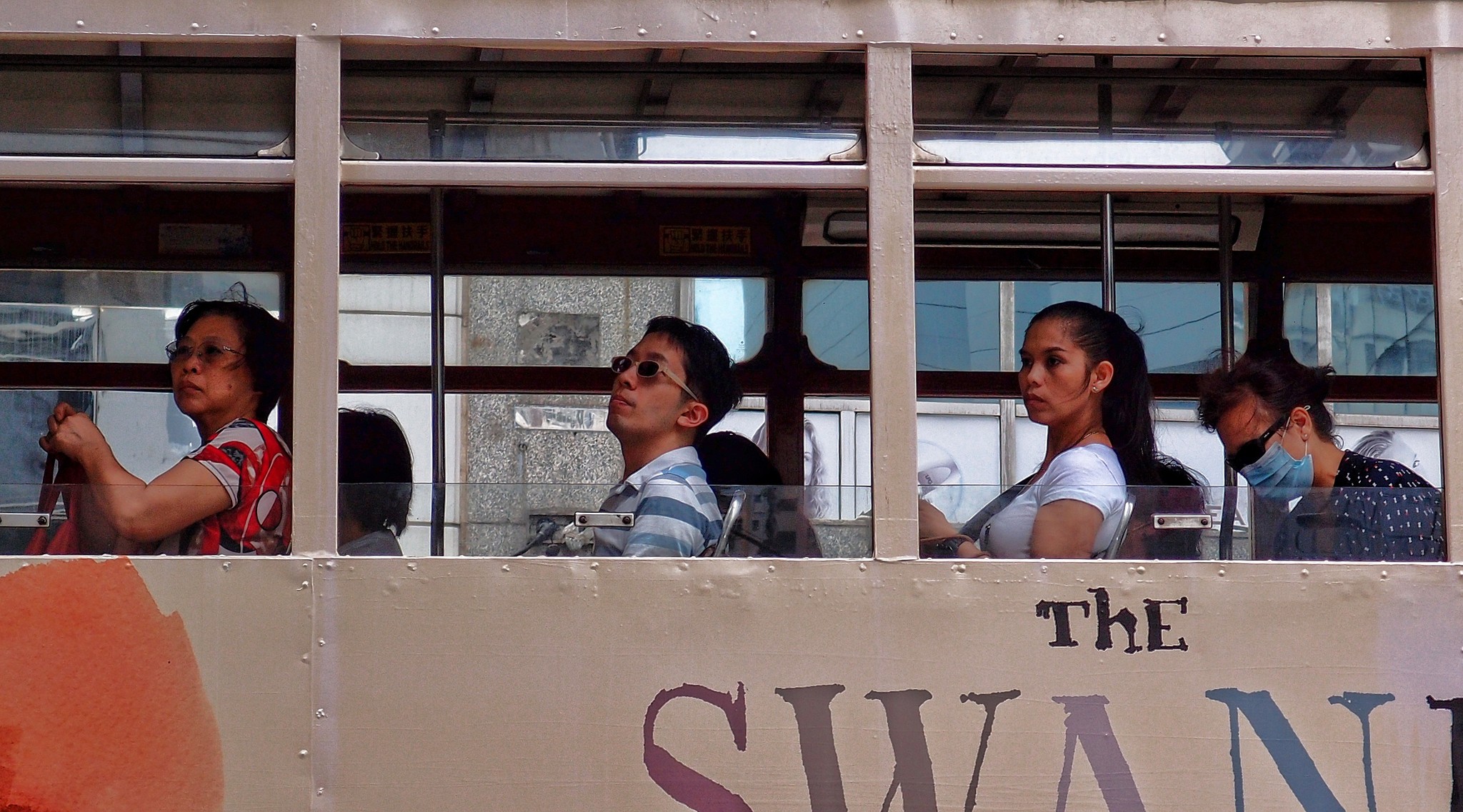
[590,315,746,558]
[916,301,1159,560]
[697,429,822,559]
[1196,334,1447,562]
[38,280,292,557]
[338,403,417,556]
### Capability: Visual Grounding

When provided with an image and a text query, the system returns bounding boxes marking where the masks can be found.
[1234,405,1315,504]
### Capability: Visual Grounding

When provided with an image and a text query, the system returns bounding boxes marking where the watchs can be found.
[933,537,965,558]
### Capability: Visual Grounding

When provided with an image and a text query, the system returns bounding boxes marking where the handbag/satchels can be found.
[21,438,80,555]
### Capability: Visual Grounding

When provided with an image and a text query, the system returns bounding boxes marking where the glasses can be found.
[164,339,247,364]
[611,355,699,404]
[1224,412,1287,474]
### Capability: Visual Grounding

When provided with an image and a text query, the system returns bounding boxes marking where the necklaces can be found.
[1041,430,1107,471]
